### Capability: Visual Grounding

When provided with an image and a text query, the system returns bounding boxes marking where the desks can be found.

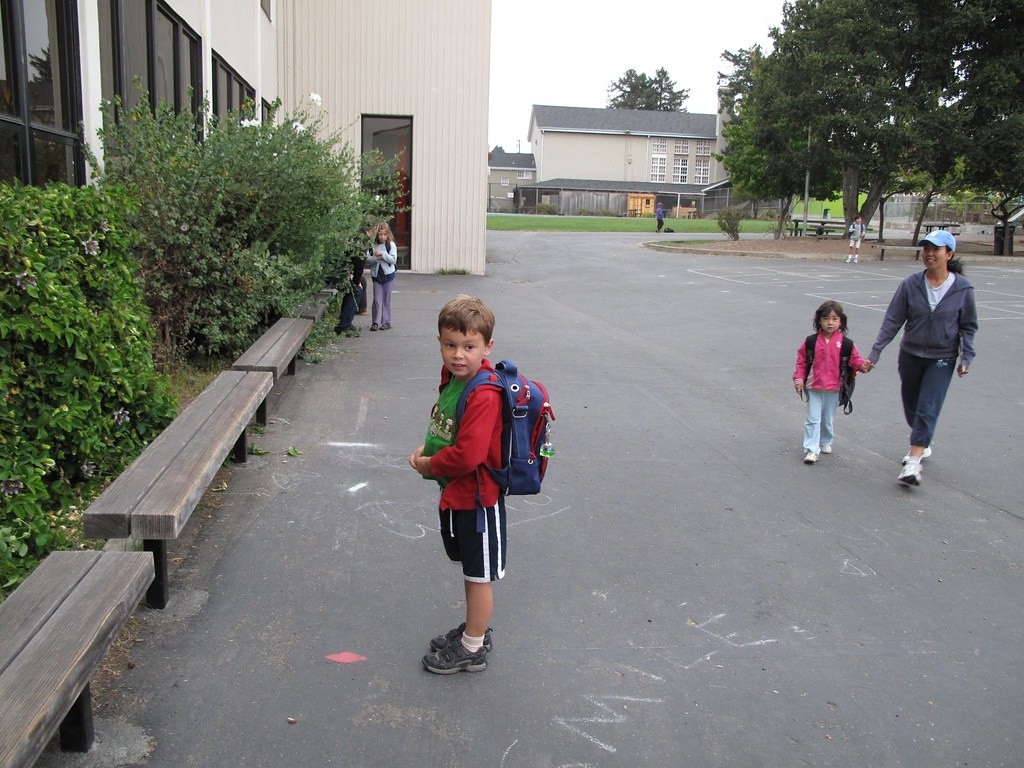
[792,219,845,236]
[922,223,960,237]
[687,211,701,220]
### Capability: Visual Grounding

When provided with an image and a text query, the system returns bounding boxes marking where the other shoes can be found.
[361,308,369,314]
[370,323,378,331]
[380,323,391,330]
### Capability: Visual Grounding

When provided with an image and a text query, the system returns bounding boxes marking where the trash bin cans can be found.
[995,225,1014,256]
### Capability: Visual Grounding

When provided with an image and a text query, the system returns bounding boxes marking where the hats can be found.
[918,230,956,252]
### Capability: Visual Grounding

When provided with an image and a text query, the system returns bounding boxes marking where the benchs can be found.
[870,244,923,261]
[232,317,313,427]
[0,550,155,768]
[295,288,338,322]
[810,224,873,231]
[910,232,960,236]
[786,227,835,235]
[85,370,274,609]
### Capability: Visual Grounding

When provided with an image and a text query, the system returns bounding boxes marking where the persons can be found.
[410,294,506,674]
[334,215,380,337]
[794,302,871,464]
[845,214,866,263]
[367,223,397,331]
[656,203,664,232]
[864,231,978,487]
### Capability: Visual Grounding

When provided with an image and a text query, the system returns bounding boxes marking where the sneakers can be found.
[822,445,832,453]
[898,460,921,487]
[845,258,858,263]
[431,623,493,653]
[422,636,489,675]
[803,452,819,463]
[902,445,931,464]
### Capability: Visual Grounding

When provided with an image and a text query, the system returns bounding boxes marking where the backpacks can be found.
[849,232,853,238]
[804,333,857,407]
[455,360,555,497]
